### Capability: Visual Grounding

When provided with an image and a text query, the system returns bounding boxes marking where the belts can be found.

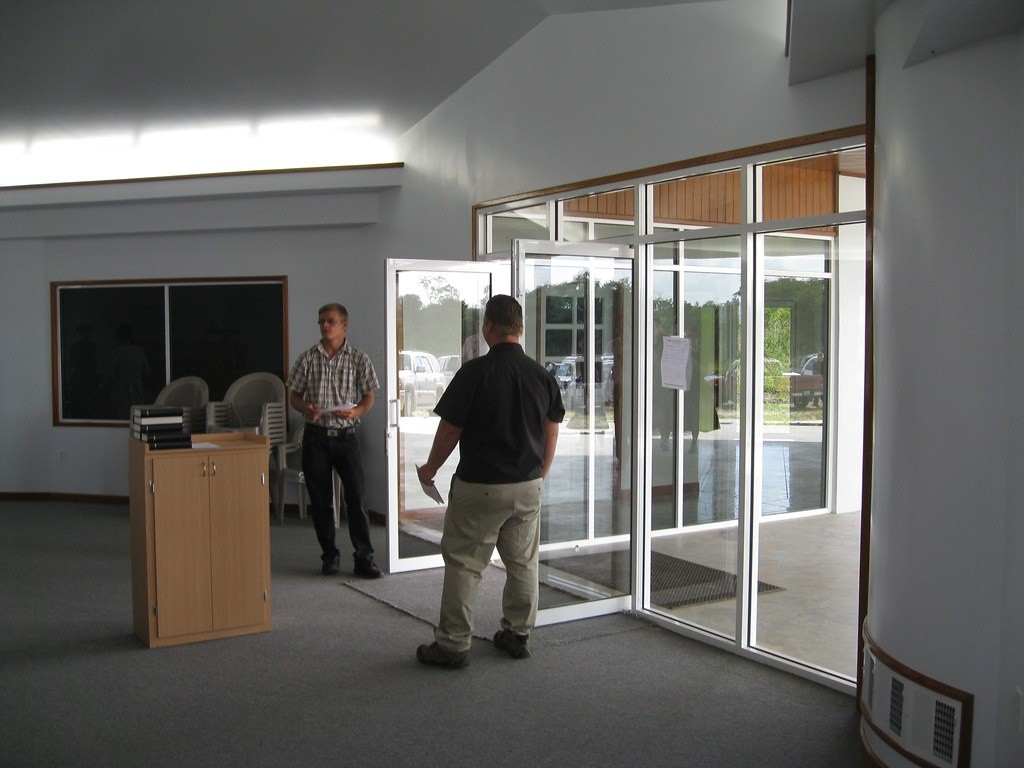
[304,425,355,437]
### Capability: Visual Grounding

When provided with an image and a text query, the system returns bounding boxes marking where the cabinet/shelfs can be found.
[129,431,272,649]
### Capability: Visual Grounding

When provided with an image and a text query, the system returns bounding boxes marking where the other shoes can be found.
[493,630,532,660]
[321,555,340,574]
[353,560,384,579]
[416,640,469,669]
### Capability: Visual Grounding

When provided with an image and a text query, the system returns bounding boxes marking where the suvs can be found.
[439,354,462,388]
[398,350,448,418]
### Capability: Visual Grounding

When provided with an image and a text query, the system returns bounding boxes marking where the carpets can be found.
[541,547,786,609]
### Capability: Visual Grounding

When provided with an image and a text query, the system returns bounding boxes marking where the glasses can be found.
[318,318,344,324]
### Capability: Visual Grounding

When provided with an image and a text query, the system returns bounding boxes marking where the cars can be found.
[544,353,615,389]
[703,349,826,415]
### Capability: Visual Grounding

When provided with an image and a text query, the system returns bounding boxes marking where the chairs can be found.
[277,423,341,528]
[205,372,287,455]
[129,376,209,434]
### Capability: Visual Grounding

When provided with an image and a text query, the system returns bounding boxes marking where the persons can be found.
[610,314,721,468]
[286,304,387,579]
[812,352,824,407]
[417,295,566,666]
